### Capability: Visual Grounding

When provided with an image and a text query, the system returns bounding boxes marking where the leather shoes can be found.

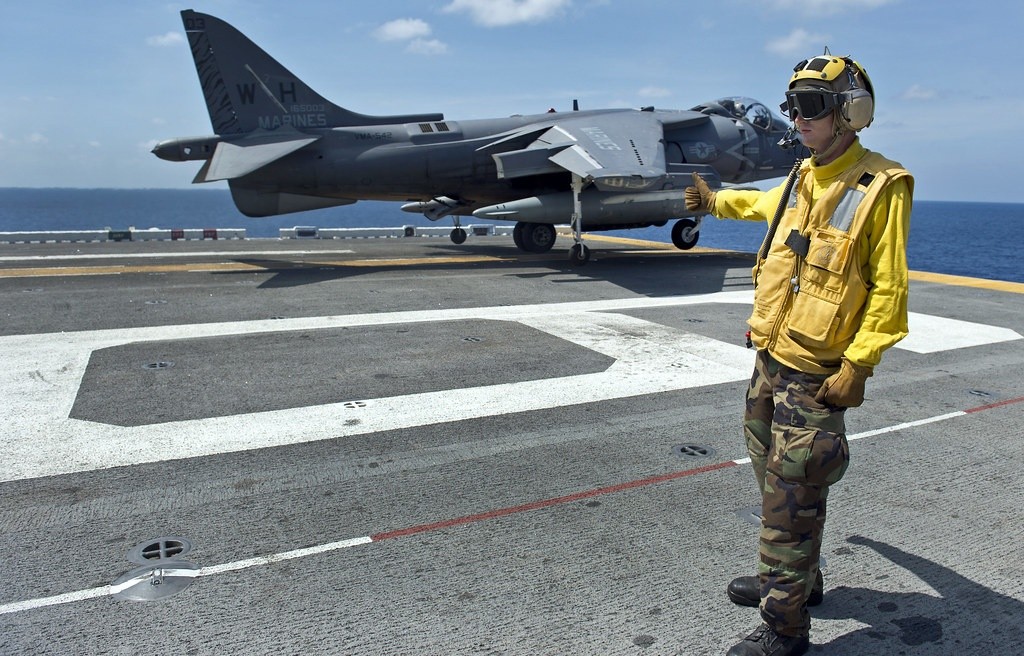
[727,564,823,607]
[725,622,810,656]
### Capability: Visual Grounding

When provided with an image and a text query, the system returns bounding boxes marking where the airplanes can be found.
[150,8,811,266]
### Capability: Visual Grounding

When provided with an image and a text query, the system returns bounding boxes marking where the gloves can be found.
[685,171,717,213]
[814,358,873,407]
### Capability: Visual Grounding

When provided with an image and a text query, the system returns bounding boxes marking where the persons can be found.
[735,103,749,121]
[684,54,914,656]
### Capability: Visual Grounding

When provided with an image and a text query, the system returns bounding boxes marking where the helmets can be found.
[789,55,876,131]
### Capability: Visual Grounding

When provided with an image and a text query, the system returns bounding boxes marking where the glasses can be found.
[785,90,833,122]
